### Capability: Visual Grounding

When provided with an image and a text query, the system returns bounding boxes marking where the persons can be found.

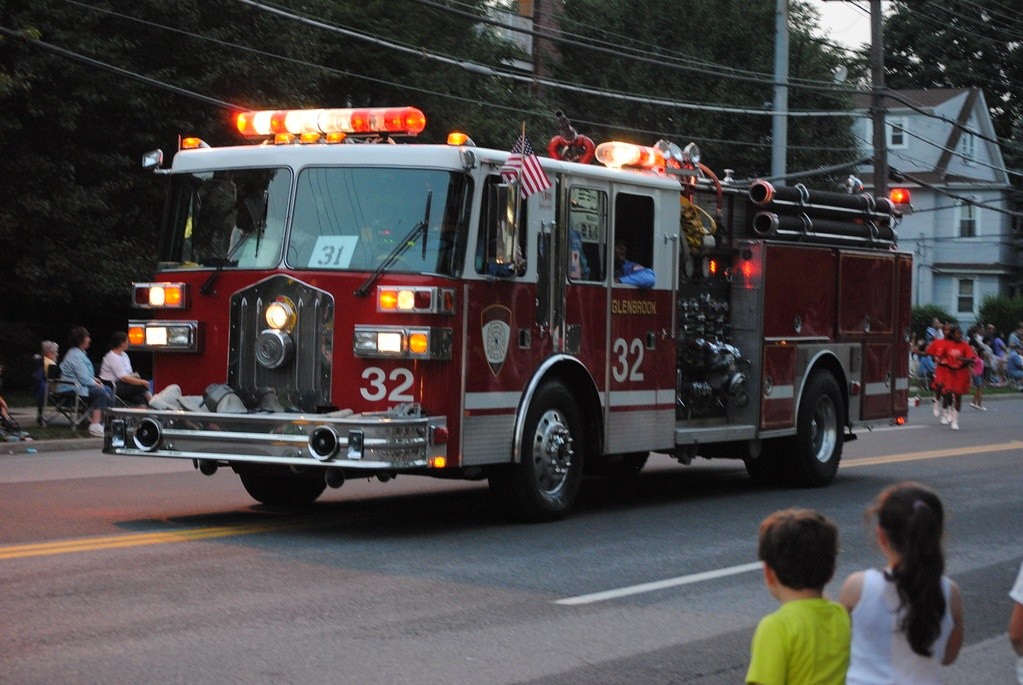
[97,331,152,406]
[614,238,656,289]
[837,481,963,685]
[743,506,853,685]
[912,323,954,424]
[971,345,987,412]
[54,326,113,438]
[40,341,62,380]
[937,325,978,431]
[910,319,1023,391]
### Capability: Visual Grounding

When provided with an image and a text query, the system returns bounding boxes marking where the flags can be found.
[502,131,552,199]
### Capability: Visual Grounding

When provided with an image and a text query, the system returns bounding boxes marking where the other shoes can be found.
[90,431,104,438]
[89,422,104,435]
[977,405,987,410]
[970,402,980,409]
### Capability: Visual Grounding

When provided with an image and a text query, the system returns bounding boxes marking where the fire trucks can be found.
[101,105,914,524]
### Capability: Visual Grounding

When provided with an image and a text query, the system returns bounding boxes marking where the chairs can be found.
[32,354,129,434]
[912,363,931,390]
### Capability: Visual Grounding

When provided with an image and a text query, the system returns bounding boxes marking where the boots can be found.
[947,405,952,421]
[940,407,948,423]
[934,398,940,416]
[951,408,960,429]
